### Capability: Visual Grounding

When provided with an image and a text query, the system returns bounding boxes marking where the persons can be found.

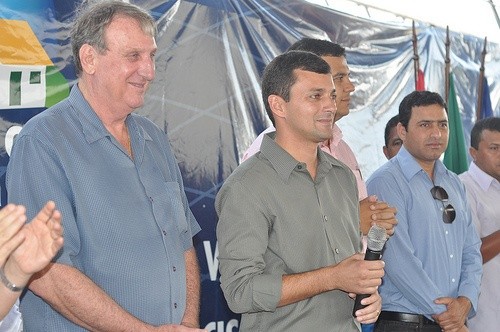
[366,91,482,332]
[457,116,500,332]
[214,50,386,332]
[0,200,66,332]
[381,115,403,161]
[5,0,206,332]
[241,36,397,240]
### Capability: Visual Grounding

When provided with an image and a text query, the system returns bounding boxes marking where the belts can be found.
[379,310,440,326]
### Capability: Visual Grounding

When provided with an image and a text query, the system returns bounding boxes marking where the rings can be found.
[376,309,380,316]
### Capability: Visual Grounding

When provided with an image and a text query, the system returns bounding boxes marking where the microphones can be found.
[353,225,387,317]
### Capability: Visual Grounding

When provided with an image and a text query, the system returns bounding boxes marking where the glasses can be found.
[429,186,456,224]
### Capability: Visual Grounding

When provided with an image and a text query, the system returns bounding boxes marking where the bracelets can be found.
[0,272,25,292]
